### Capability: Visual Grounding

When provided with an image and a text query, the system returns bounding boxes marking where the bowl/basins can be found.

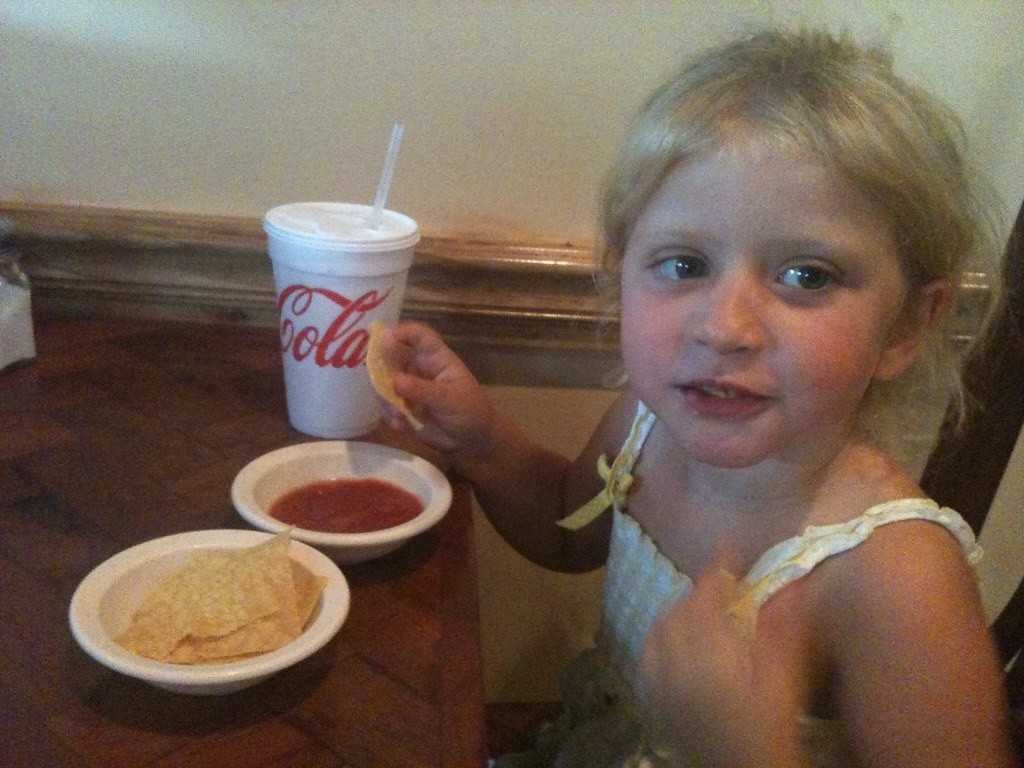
[230,441,453,564]
[68,530,350,696]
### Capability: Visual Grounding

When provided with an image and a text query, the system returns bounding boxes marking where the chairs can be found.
[920,187,1023,768]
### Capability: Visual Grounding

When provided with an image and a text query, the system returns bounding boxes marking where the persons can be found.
[376,25,1017,768]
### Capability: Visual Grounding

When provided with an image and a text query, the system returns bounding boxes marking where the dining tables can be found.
[1,318,486,766]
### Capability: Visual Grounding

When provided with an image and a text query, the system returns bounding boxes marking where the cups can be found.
[263,201,419,441]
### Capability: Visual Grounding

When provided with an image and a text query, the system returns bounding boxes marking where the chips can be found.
[113,524,327,665]
